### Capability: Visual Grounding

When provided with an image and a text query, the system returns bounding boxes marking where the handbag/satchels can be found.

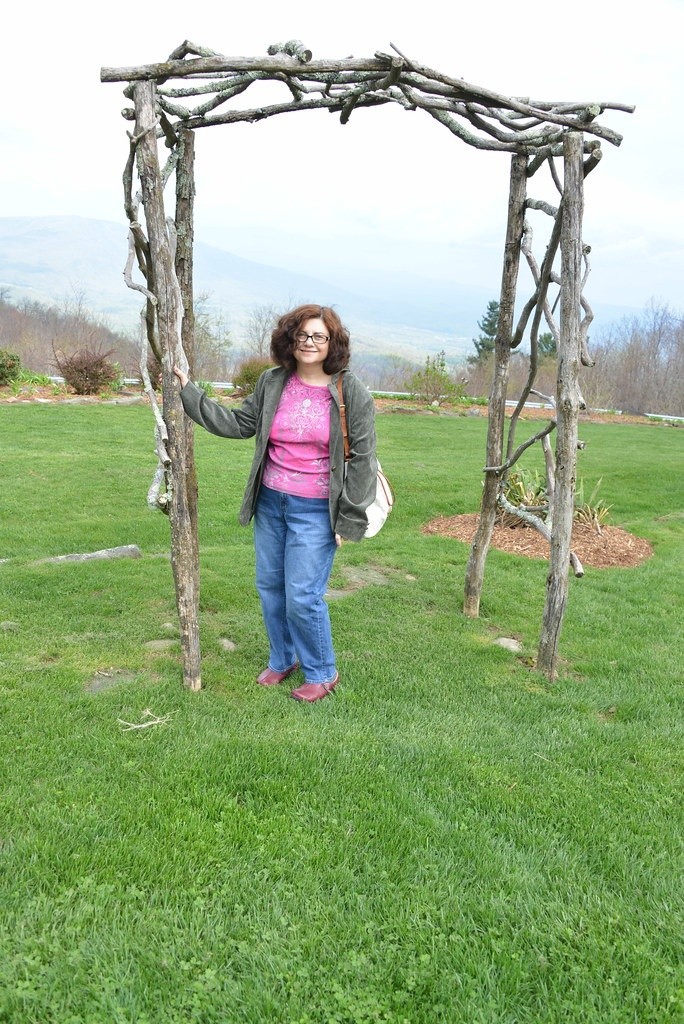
[338,372,395,538]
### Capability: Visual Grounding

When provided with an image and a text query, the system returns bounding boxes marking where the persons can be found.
[158,303,376,703]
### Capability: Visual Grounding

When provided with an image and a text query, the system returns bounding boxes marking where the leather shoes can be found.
[257,662,297,686]
[291,670,338,702]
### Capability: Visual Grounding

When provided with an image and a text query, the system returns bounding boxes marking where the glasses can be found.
[297,331,331,344]
